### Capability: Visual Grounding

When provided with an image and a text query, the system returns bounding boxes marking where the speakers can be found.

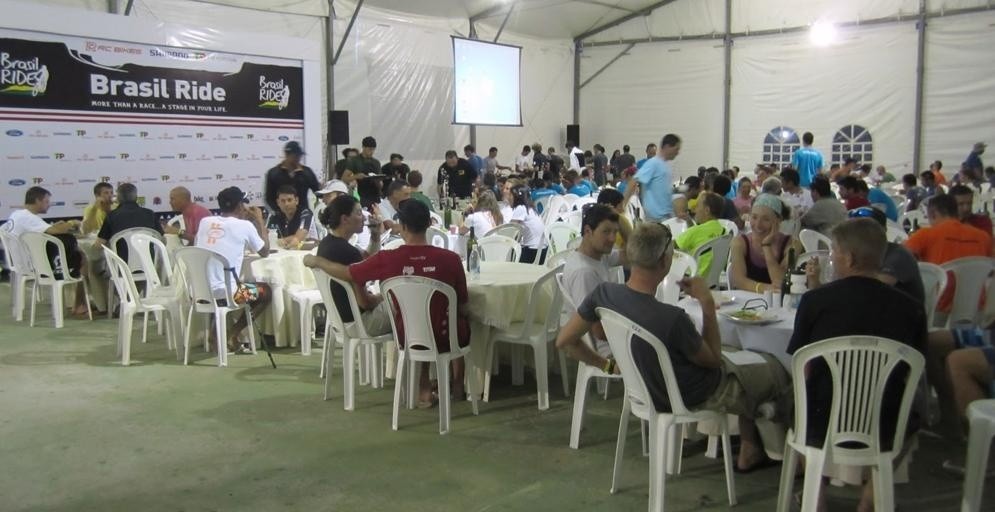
[328,110,349,145]
[567,124,579,148]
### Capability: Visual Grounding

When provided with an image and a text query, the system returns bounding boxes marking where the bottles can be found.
[765,248,833,310]
[908,218,919,236]
[268,224,279,251]
[466,226,481,276]
[673,176,682,193]
[440,186,459,229]
[718,269,727,292]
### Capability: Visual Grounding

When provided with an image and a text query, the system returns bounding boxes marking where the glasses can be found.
[846,207,875,218]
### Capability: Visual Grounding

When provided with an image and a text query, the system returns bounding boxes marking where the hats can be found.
[313,177,350,195]
[283,140,306,156]
[215,185,248,205]
[972,141,988,147]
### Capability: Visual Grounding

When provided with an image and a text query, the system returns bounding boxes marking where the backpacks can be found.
[39,231,81,279]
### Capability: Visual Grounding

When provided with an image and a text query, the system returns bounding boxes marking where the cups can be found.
[449,225,457,234]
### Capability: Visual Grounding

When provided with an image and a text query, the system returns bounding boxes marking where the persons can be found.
[0,128,994,511]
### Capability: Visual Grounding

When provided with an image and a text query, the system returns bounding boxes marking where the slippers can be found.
[224,343,254,356]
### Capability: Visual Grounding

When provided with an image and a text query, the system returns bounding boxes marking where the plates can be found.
[713,293,781,324]
[366,174,388,178]
[466,274,496,285]
